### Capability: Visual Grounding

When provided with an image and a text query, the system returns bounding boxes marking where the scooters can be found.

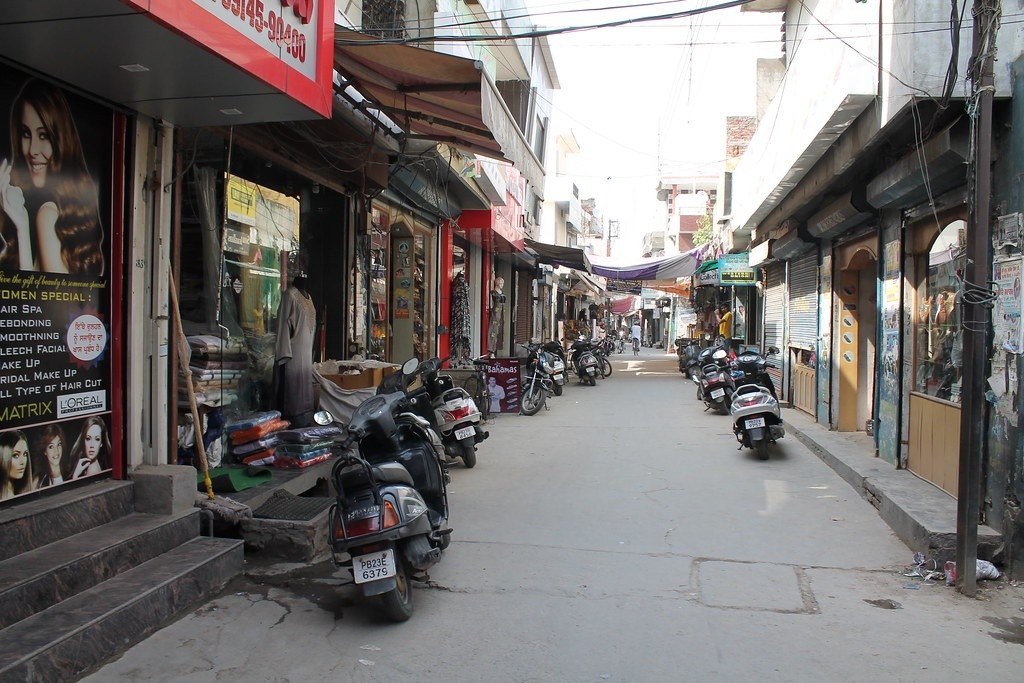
[697,343,736,415]
[713,345,785,460]
[675,334,709,386]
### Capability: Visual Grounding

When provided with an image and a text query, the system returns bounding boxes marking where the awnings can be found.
[524,237,592,276]
[334,23,546,201]
[576,272,605,295]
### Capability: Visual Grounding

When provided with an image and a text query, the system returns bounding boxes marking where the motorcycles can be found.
[520,336,570,416]
[597,331,627,356]
[313,352,489,621]
[570,340,599,385]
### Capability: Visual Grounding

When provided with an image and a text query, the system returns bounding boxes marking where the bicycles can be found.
[589,340,612,380]
[463,355,495,422]
[631,337,641,356]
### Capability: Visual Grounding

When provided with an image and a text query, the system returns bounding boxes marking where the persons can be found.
[272,273,316,426]
[0,78,104,275]
[631,322,641,351]
[69,416,112,479]
[929,290,966,398]
[33,424,70,489]
[714,305,732,354]
[580,308,587,321]
[487,277,506,358]
[0,430,33,499]
[452,273,471,341]
